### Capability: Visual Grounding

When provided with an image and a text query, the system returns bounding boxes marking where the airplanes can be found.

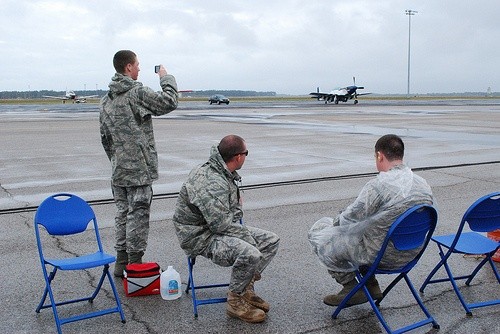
[41,89,99,104]
[308,76,373,105]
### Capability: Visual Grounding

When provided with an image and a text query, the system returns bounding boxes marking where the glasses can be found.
[233,150,248,158]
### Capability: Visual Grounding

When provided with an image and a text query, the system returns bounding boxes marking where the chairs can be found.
[34,193,126,334]
[419,192,500,316]
[185,219,255,318]
[331,203,440,334]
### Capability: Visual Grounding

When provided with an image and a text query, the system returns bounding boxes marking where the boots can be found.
[243,276,268,311]
[323,282,367,305]
[129,253,143,264]
[366,279,382,299]
[114,251,128,276]
[226,293,266,321]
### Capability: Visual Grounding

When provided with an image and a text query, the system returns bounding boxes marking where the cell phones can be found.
[155,66,160,73]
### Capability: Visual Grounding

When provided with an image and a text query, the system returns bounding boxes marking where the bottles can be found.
[159,266,182,300]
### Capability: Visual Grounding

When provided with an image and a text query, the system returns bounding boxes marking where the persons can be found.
[305,133,437,307]
[172,135,280,324]
[96,49,179,280]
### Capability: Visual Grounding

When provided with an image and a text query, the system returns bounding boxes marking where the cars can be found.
[208,94,230,105]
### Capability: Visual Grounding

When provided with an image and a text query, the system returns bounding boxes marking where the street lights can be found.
[405,9,418,99]
